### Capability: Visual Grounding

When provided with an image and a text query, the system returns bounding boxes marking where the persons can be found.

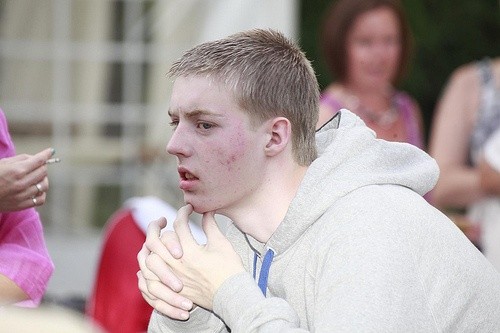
[137,0,500,333]
[0,97,110,333]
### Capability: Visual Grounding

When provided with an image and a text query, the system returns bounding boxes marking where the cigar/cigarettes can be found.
[45,158,61,163]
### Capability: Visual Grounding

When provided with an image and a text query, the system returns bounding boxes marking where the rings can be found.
[32,199,37,206]
[35,185,43,195]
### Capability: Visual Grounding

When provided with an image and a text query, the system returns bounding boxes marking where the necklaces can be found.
[334,84,400,128]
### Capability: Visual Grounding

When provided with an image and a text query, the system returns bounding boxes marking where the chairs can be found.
[82,207,154,333]
